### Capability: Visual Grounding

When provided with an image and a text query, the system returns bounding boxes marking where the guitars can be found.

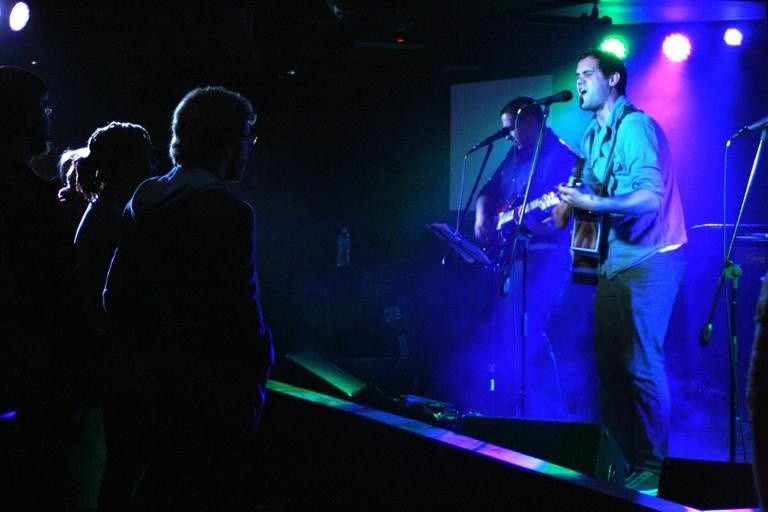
[570,159,605,285]
[490,191,562,247]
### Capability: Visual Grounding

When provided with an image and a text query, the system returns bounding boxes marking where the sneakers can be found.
[624,470,658,496]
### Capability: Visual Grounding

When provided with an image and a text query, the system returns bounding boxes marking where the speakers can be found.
[458,419,614,486]
[656,457,760,510]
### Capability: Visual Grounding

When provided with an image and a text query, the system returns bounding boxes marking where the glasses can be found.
[42,103,57,117]
[245,133,258,148]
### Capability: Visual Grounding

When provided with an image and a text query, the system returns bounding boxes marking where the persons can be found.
[473,97,598,418]
[511,51,688,494]
[1,66,274,507]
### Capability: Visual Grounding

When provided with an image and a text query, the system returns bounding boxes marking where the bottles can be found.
[336,228,351,267]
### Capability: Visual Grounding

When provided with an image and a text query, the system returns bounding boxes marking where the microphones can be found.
[526,90,573,105]
[467,125,509,155]
[736,115,767,135]
